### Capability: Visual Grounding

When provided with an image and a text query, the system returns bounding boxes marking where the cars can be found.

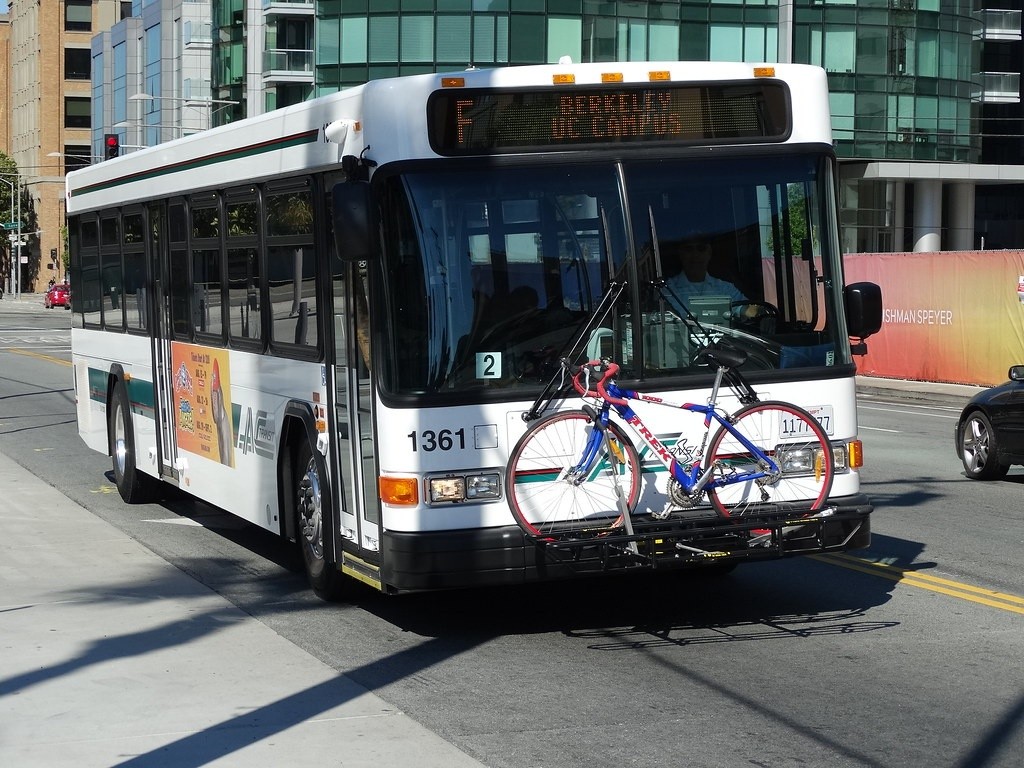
[43,283,71,309]
[61,286,71,310]
[954,364,1024,483]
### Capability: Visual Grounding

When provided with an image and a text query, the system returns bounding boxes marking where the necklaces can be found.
[693,282,705,297]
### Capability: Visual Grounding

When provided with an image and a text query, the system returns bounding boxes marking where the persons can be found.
[48,275,68,289]
[655,233,773,333]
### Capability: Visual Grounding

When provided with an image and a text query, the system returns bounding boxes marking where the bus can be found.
[63,56,883,599]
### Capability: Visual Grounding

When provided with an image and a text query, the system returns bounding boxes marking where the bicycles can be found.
[501,352,832,547]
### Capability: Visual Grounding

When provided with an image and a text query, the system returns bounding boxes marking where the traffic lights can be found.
[104,133,119,161]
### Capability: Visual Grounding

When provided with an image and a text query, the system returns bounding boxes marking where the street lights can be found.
[129,93,240,131]
[112,116,207,139]
